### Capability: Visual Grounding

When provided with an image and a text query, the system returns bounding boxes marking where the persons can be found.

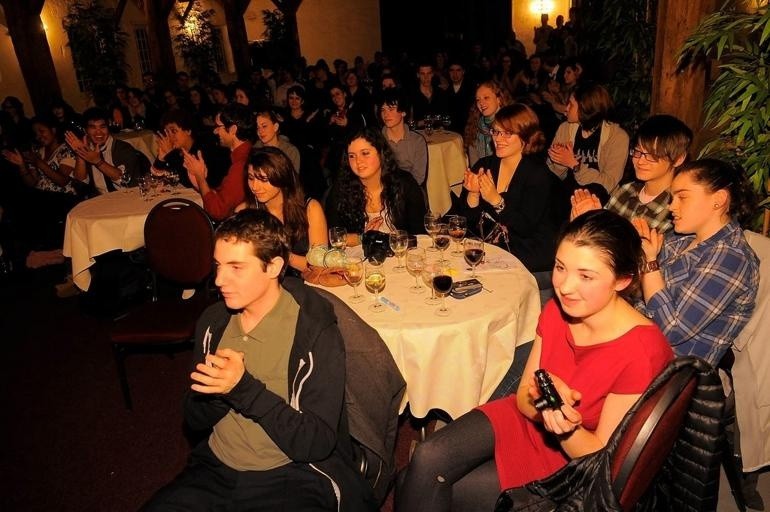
[0,11,629,308]
[137,207,376,511]
[530,114,693,308]
[394,208,674,512]
[489,157,764,404]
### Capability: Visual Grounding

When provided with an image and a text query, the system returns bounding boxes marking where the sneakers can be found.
[181,286,196,300]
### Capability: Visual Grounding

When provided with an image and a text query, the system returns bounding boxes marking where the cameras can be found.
[534,369,567,419]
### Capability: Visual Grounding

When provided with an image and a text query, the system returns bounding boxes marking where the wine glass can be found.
[110,119,146,137]
[118,173,182,202]
[407,114,453,142]
[305,213,485,317]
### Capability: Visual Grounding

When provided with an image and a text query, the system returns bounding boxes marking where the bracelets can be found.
[639,260,660,273]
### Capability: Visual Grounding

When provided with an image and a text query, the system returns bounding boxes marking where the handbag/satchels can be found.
[302,248,353,287]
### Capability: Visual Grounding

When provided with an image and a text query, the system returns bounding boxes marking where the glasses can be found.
[489,129,517,137]
[629,147,667,164]
